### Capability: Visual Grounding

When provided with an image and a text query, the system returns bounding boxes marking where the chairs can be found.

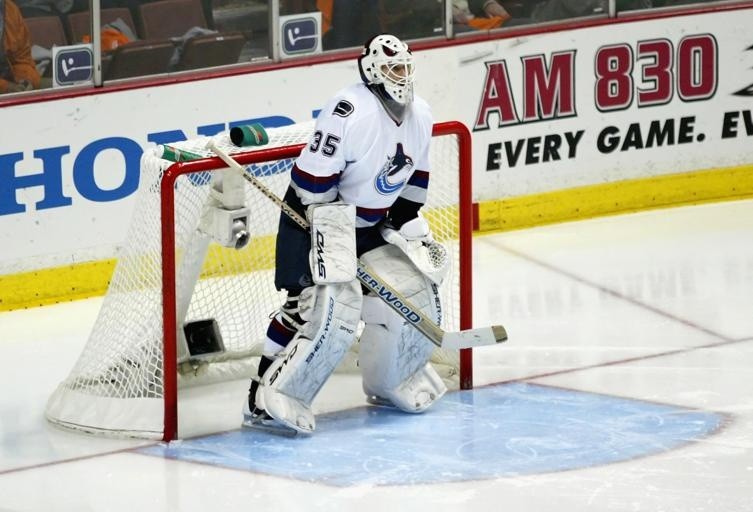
[0,1,246,82]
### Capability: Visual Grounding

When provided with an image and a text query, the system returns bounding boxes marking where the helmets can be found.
[356,32,417,105]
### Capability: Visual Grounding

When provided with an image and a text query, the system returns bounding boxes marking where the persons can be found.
[0,0,53,95]
[313,0,691,51]
[240,31,452,439]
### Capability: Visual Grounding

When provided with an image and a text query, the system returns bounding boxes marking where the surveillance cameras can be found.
[104,317,227,387]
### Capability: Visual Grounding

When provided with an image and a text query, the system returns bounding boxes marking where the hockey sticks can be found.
[206,136,507,350]
